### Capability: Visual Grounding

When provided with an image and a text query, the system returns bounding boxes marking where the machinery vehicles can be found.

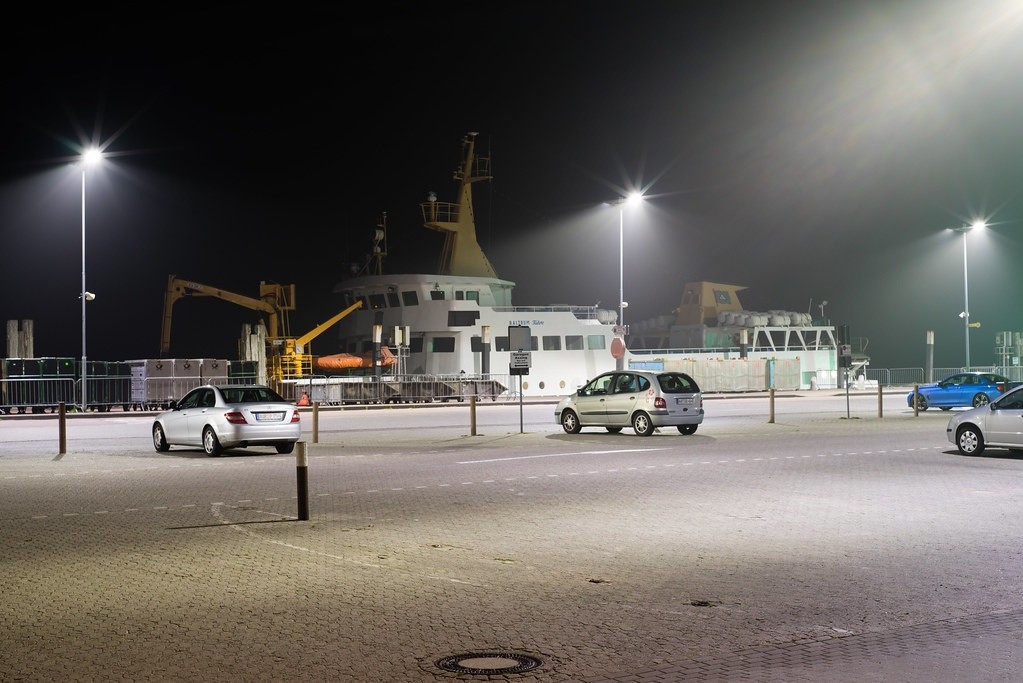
[160,277,362,403]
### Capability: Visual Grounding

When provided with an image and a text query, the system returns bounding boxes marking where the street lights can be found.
[81,148,103,413]
[961,220,983,372]
[616,193,642,386]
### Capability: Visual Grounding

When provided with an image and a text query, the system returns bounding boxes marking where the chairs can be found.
[204,393,216,406]
[668,378,676,390]
[618,376,634,391]
[240,391,258,402]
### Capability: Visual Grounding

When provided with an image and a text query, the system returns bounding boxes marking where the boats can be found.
[296,131,880,395]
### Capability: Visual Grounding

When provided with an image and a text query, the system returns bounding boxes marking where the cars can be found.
[152,384,300,457]
[947,384,1023,457]
[907,372,1023,412]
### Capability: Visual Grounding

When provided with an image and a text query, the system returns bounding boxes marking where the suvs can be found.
[554,370,705,437]
